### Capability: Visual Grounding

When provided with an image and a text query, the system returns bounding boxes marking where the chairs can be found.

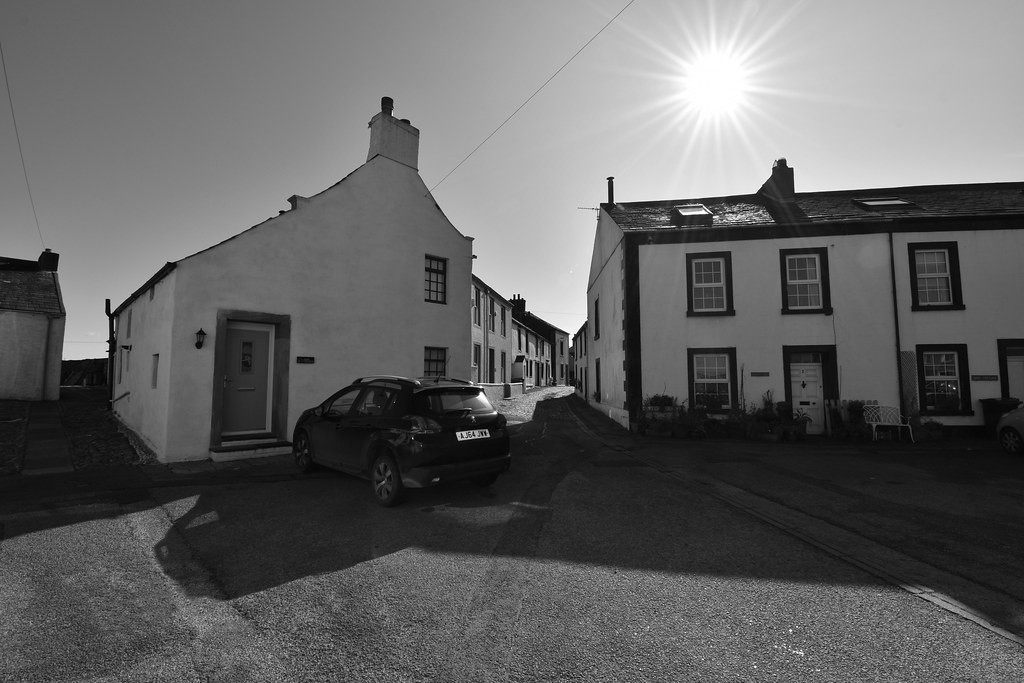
[363,391,387,416]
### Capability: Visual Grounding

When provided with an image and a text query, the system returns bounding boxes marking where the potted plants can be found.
[628,389,813,443]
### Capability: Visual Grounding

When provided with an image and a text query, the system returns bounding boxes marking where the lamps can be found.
[194,328,207,349]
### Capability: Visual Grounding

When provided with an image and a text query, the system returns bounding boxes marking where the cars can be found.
[996,406,1024,453]
[292,356,512,507]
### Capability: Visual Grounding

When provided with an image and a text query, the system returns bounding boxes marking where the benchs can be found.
[862,404,916,444]
